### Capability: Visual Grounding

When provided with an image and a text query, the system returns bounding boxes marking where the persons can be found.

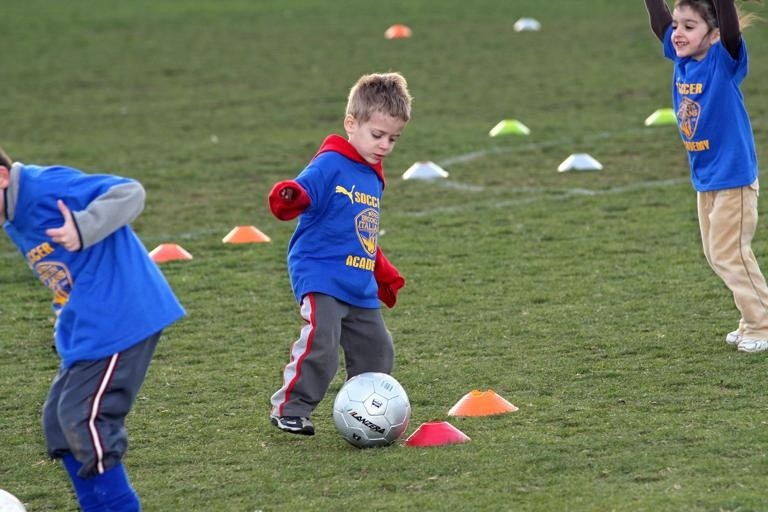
[264,70,420,436]
[0,148,191,511]
[643,0,767,356]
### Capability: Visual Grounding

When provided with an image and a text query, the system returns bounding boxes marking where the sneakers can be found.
[271,410,316,435]
[725,328,767,353]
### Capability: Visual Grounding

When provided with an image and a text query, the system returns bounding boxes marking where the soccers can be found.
[332,373,411,449]
[1,489,27,511]
